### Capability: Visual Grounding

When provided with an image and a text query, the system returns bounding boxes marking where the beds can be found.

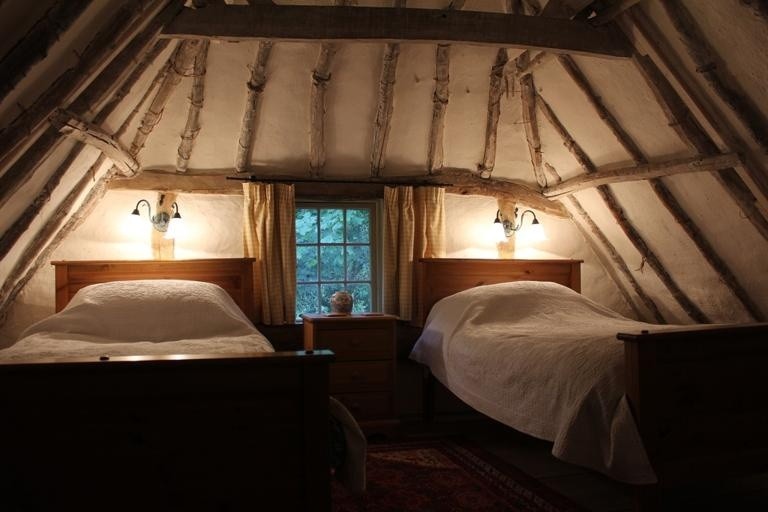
[407,259,767,512]
[0,257,372,512]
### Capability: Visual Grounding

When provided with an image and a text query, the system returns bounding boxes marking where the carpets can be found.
[323,434,593,512]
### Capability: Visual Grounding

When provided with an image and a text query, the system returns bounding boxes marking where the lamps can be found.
[130,191,192,232]
[492,199,540,239]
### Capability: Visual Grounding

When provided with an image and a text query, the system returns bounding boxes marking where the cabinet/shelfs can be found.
[299,312,399,445]
